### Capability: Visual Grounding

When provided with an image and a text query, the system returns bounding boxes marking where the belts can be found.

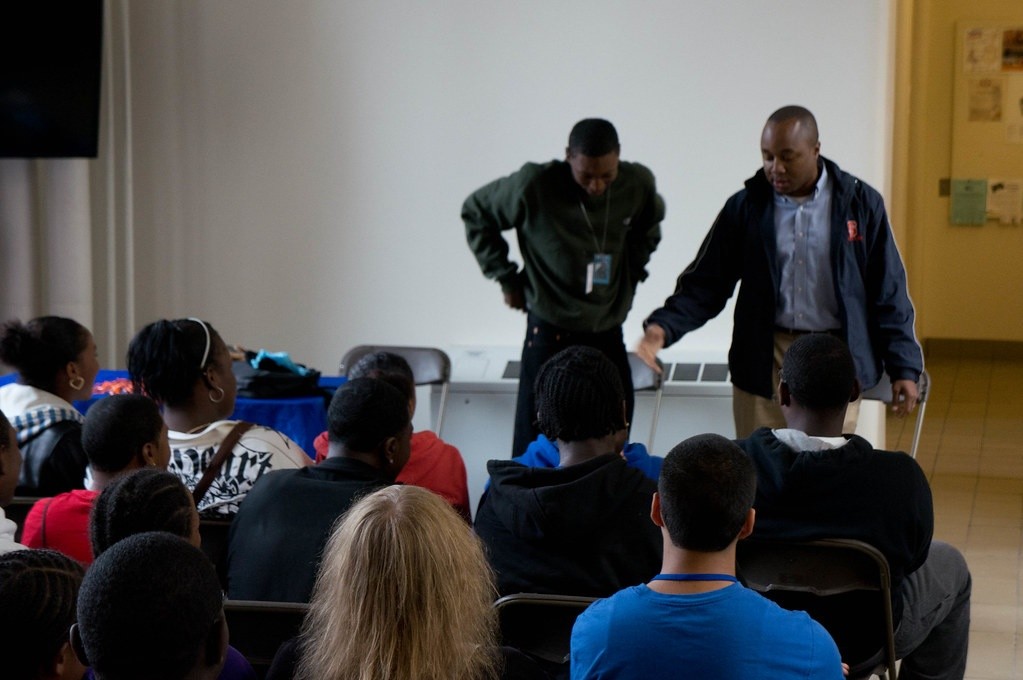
[774,325,839,338]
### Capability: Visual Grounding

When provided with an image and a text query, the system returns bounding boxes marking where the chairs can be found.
[736,538,897,680]
[853,369,930,457]
[223,600,309,680]
[628,351,665,457]
[339,343,452,439]
[492,591,600,680]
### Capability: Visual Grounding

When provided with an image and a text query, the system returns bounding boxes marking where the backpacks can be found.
[224,344,334,415]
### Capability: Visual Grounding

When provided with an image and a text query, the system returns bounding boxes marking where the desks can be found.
[0,367,347,463]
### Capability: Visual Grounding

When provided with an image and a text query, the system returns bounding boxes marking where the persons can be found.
[461,117,665,460]
[0,313,972,680]
[634,106,925,439]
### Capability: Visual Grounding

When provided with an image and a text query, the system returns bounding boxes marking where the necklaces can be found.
[185,422,210,435]
